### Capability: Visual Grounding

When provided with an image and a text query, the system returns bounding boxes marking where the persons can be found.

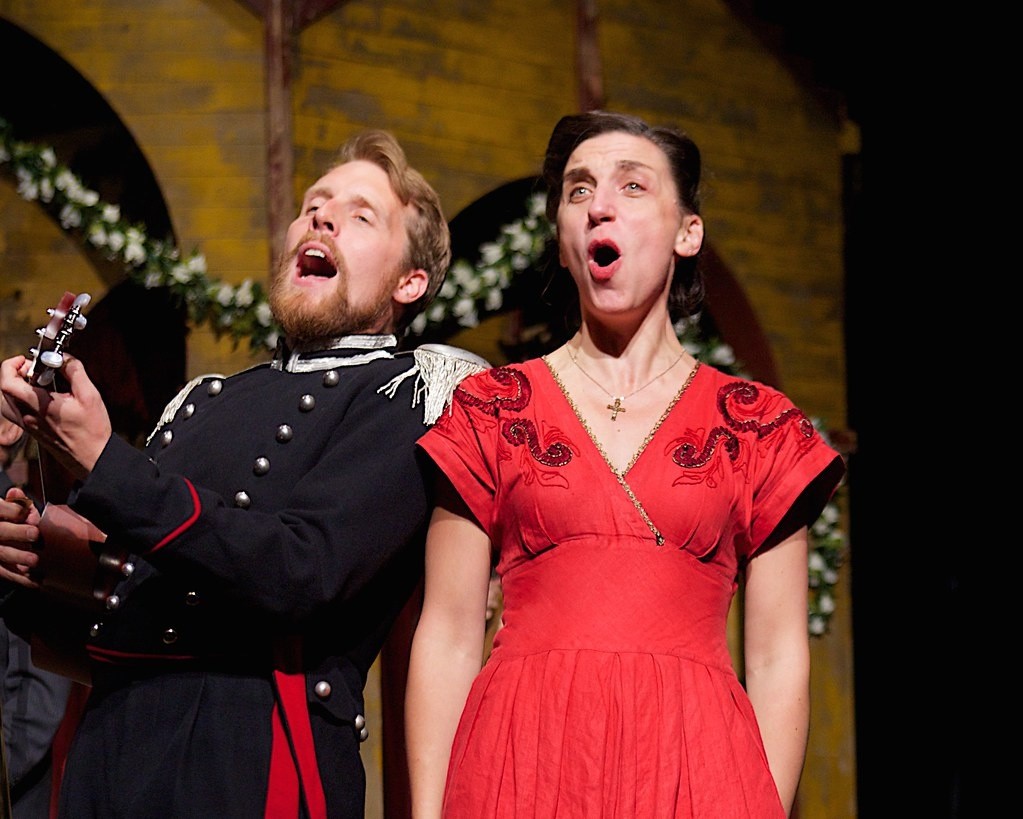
[1,130,491,819]
[405,110,813,819]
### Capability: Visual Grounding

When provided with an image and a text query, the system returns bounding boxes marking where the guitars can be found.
[18,290,105,677]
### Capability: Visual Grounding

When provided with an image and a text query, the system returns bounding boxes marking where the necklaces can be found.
[566,338,687,423]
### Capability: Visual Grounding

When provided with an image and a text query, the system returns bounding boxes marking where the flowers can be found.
[11,143,851,637]
